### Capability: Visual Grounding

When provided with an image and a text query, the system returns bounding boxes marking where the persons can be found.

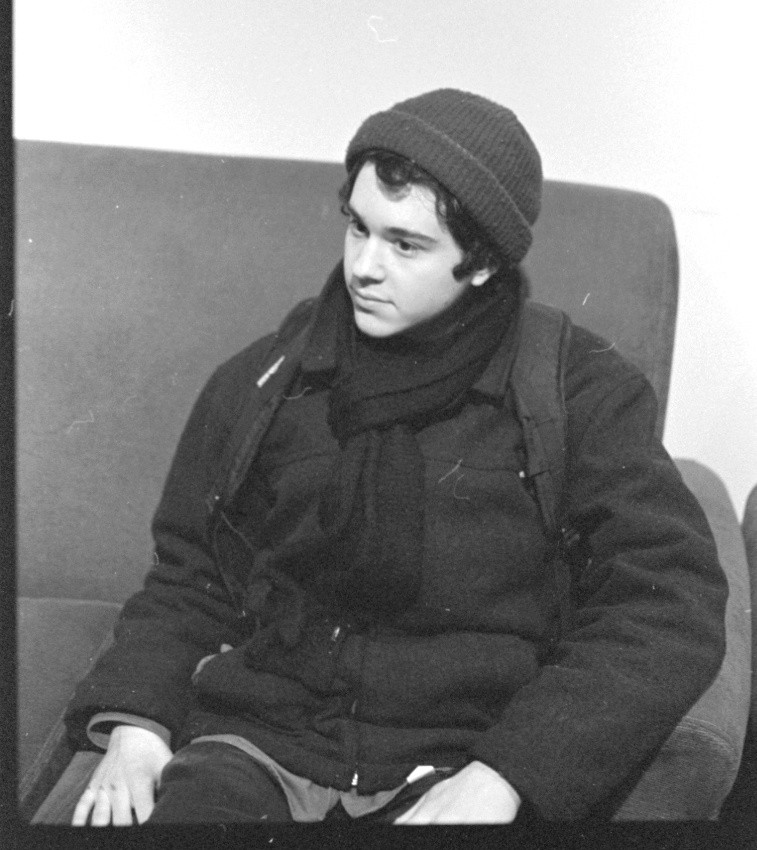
[63,86,731,825]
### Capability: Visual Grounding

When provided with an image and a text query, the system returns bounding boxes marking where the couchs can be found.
[17,135,751,824]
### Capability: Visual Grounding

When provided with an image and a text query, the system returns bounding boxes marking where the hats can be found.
[346,83,543,261]
